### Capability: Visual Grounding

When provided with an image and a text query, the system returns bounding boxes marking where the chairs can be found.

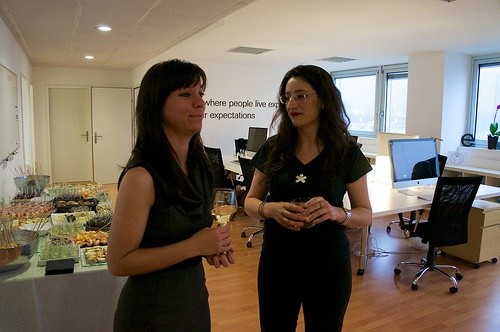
[394,176,484,294]
[203,146,267,247]
[386,154,448,231]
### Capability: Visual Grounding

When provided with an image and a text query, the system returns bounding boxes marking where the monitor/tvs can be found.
[246,127,268,152]
[389,138,441,196]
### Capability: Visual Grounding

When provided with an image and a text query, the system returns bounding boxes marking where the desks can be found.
[222,153,260,177]
[342,155,500,275]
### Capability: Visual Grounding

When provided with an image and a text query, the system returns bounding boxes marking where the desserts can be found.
[0,193,113,266]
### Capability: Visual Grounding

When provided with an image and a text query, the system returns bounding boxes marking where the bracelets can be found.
[258,201,266,219]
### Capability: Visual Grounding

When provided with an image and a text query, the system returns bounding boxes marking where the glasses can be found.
[279,90,317,105]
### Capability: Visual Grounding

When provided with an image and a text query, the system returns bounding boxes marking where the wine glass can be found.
[212,188,234,260]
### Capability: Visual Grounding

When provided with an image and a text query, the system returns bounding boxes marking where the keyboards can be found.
[245,151,257,157]
[417,193,434,201]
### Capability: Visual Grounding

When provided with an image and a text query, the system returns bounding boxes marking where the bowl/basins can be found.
[14,175,50,196]
[0,230,40,272]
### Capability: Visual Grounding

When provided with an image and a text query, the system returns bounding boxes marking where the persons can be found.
[243,65,373,332]
[107,58,236,332]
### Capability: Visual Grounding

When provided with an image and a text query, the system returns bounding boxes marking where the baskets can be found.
[0,245,24,264]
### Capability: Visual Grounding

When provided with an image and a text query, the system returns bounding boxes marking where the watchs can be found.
[339,208,352,227]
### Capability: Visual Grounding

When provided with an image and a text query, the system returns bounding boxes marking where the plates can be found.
[0,185,114,276]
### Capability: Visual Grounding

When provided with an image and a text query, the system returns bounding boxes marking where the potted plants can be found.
[488,122,500,150]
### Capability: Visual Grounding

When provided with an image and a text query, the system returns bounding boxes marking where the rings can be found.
[319,201,323,208]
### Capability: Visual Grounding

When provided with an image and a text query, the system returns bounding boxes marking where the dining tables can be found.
[0,180,129,332]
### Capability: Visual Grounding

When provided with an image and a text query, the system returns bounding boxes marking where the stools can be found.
[441,200,500,268]
